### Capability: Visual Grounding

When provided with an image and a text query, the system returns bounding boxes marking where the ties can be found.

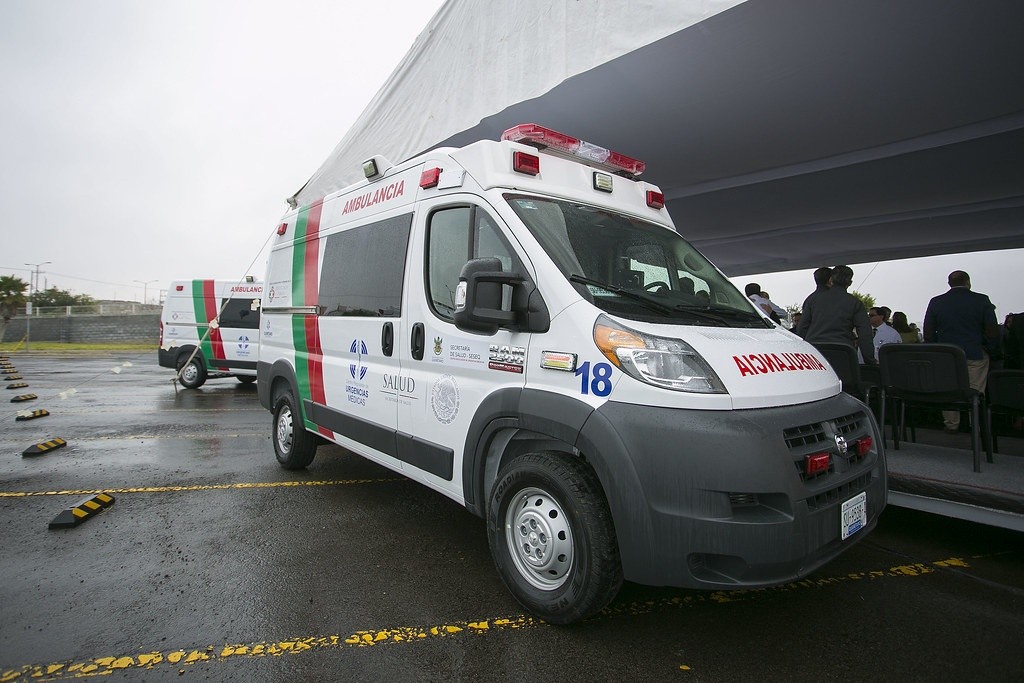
[872,328,877,339]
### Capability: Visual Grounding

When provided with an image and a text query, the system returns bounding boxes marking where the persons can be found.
[678,277,710,304]
[745,283,788,326]
[789,264,877,394]
[922,270,1002,434]
[857,306,923,366]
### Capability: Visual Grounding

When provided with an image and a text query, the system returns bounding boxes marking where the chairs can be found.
[811,341,1024,472]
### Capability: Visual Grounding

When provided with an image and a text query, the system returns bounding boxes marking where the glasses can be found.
[848,281,853,286]
[869,313,877,318]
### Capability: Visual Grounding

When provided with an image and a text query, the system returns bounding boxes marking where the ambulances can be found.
[157,273,262,389]
[255,124,889,627]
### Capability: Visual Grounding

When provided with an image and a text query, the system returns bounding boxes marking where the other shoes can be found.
[968,389,983,400]
[944,426,959,434]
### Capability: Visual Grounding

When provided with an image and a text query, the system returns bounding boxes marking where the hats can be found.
[745,283,765,294]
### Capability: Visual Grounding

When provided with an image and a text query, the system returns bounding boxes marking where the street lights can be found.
[25,261,52,292]
[134,279,160,304]
